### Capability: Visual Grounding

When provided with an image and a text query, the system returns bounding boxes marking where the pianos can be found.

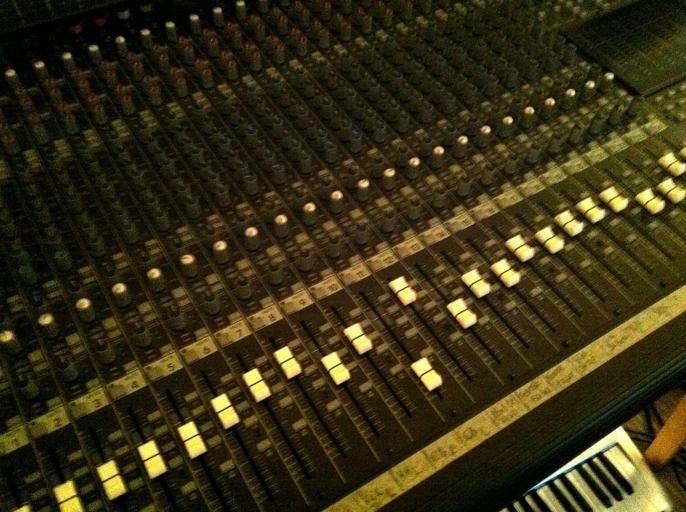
[497,425,674,511]
